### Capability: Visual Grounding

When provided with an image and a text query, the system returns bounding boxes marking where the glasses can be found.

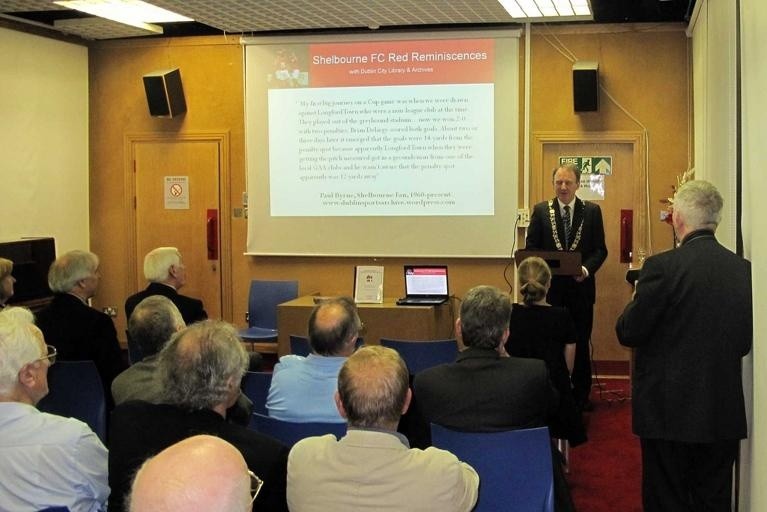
[247,471,264,505]
[33,345,57,365]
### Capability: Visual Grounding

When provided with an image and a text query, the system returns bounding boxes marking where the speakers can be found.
[143,67,186,120]
[572,60,601,113]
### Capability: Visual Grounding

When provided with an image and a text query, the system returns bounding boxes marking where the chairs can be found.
[379,337,460,377]
[429,422,556,512]
[241,366,269,409]
[234,278,299,354]
[33,358,106,443]
[291,334,362,361]
[251,411,343,450]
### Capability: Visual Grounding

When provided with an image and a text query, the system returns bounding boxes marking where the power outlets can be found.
[516,209,530,227]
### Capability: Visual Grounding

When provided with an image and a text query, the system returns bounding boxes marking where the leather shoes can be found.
[575,394,591,410]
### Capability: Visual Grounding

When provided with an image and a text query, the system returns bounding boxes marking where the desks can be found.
[276,290,456,360]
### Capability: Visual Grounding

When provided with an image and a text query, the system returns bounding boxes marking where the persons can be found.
[266,257,577,511]
[614,179,752,512]
[526,163,608,412]
[1,246,264,512]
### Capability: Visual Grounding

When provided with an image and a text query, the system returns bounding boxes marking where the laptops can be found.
[396,264,450,308]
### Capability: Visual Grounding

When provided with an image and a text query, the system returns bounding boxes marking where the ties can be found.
[563,205,571,252]
